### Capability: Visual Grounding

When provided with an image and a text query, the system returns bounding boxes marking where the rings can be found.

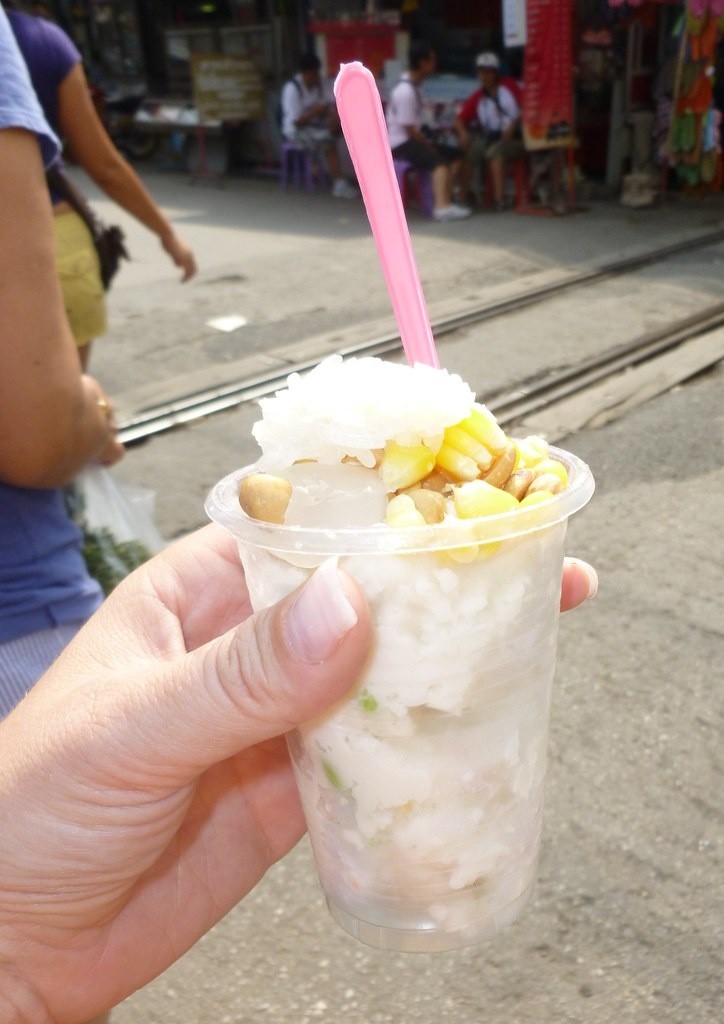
[97,400,111,414]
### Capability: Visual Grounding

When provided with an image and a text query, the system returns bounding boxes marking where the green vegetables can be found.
[63,484,155,595]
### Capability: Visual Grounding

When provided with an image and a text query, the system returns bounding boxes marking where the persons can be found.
[454,52,523,208]
[0,0,195,373]
[385,45,472,221]
[573,19,624,181]
[281,56,360,200]
[0,522,599,1024]
[0,8,123,720]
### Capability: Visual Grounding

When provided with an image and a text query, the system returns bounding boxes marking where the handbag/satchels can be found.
[64,448,167,604]
[96,227,125,291]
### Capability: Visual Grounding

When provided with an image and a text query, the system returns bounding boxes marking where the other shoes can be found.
[333,182,359,199]
[493,201,505,211]
[431,202,471,223]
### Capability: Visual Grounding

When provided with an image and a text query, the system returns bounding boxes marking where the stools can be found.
[277,141,534,215]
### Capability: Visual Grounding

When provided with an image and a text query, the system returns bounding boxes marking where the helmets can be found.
[475,53,500,68]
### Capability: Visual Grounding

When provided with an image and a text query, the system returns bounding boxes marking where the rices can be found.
[243,353,561,942]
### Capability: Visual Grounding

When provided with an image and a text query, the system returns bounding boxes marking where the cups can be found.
[203,440,595,953]
